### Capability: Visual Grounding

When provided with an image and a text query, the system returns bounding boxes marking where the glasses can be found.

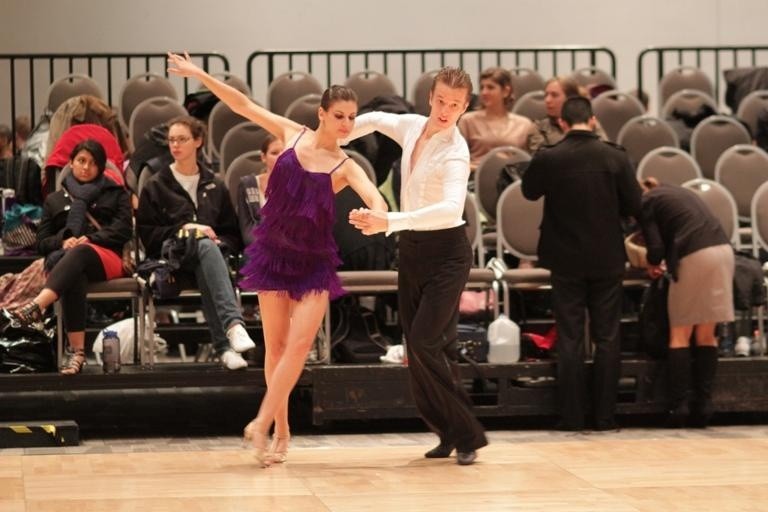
[167,134,195,144]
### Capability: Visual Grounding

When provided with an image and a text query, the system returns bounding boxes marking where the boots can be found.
[664,348,692,427]
[691,345,718,425]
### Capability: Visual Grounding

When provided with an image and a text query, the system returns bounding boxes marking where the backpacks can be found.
[333,300,392,363]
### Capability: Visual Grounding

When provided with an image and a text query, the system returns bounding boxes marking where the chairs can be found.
[45,67,768,370]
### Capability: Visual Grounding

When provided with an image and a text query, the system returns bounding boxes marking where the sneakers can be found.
[734,334,752,356]
[225,322,257,353]
[220,346,250,372]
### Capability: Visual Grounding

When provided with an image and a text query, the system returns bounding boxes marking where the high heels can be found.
[242,417,272,467]
[270,427,292,464]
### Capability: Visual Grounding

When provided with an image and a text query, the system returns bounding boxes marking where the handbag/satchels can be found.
[642,286,665,361]
[120,247,138,275]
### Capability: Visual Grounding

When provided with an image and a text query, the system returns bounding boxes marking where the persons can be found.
[0,114,44,205]
[165,49,389,465]
[337,66,489,466]
[13,139,133,374]
[629,175,737,428]
[457,68,533,190]
[237,133,285,246]
[526,76,610,156]
[521,96,643,429]
[137,116,257,370]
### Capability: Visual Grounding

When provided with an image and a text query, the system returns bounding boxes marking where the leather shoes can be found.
[452,447,476,465]
[425,435,457,457]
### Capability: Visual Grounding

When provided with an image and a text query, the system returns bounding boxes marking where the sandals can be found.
[61,350,87,374]
[13,302,45,324]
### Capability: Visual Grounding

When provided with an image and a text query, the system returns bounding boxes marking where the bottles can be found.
[99,326,123,373]
[718,322,766,358]
